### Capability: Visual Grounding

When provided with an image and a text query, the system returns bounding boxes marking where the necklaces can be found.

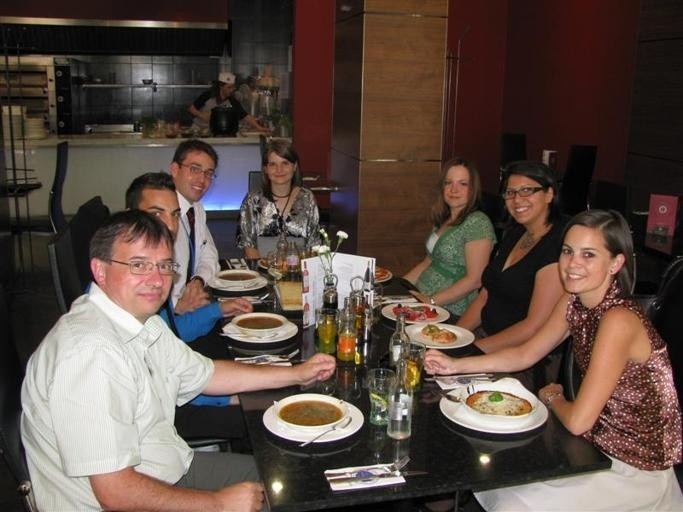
[272,189,292,217]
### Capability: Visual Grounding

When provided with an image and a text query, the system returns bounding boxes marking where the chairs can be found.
[10,138,70,282]
[45,213,238,456]
[259,135,341,231]
[4,407,43,512]
[488,131,682,299]
[68,193,110,246]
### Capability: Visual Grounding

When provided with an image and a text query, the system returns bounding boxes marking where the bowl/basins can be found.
[457,382,538,425]
[236,312,287,333]
[214,270,259,283]
[275,393,348,431]
[140,79,154,86]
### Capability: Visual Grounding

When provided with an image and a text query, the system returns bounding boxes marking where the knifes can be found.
[324,469,428,485]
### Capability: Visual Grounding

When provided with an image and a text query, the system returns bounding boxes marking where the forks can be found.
[234,347,299,365]
[216,333,281,340]
[298,415,352,451]
[315,456,411,477]
[216,292,274,304]
[426,372,498,385]
[207,282,262,291]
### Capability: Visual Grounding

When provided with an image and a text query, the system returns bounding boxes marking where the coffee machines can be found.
[245,76,282,121]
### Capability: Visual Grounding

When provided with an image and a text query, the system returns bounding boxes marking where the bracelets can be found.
[430,295,437,306]
[189,275,205,286]
[546,392,562,407]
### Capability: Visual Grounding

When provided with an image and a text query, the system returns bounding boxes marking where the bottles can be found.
[387,358,412,439]
[131,118,208,138]
[269,233,374,361]
[386,313,411,364]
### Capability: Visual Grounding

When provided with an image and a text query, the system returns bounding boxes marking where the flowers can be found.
[309,226,348,283]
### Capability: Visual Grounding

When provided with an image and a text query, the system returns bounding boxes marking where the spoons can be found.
[426,380,459,401]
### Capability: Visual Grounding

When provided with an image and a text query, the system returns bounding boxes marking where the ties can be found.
[186,206,196,284]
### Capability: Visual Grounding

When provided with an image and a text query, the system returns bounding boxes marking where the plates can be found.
[381,302,476,350]
[256,257,271,268]
[220,312,299,344]
[205,273,271,290]
[260,394,363,444]
[2,105,47,140]
[373,264,393,286]
[436,380,550,437]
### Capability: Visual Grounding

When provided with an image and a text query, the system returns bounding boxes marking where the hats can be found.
[218,72,237,85]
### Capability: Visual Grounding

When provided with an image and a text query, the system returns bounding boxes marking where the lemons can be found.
[407,363,419,386]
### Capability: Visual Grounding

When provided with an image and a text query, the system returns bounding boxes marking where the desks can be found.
[0,181,43,279]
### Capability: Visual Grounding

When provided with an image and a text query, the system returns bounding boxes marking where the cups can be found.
[367,342,425,425]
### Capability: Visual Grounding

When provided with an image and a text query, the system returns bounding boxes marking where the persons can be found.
[124,171,254,455]
[401,157,497,306]
[233,138,320,259]
[187,71,272,131]
[454,161,568,355]
[423,208,683,511]
[171,138,221,315]
[21,208,265,512]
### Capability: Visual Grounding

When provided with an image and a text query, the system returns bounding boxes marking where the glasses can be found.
[181,163,217,181]
[502,187,544,200]
[106,258,182,275]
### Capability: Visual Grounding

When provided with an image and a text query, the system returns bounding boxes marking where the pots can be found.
[210,107,238,136]
